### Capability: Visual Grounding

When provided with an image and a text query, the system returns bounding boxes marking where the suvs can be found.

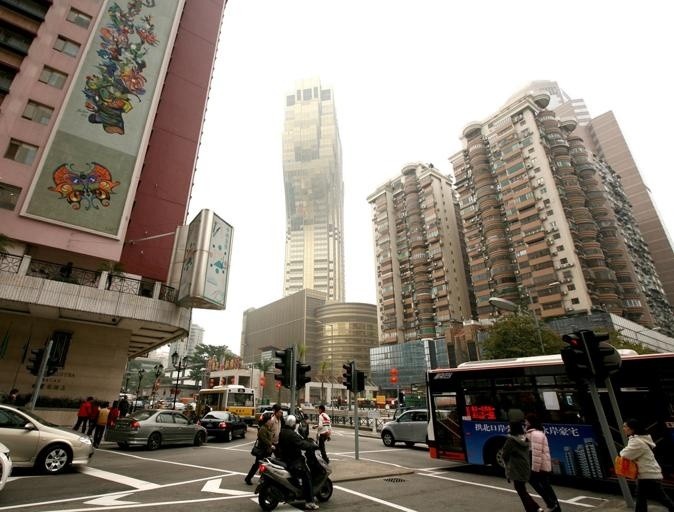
[258,410,290,436]
[248,403,287,427]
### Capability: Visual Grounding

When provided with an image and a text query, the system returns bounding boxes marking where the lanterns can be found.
[208,378,235,387]
[390,368,398,384]
[260,377,265,386]
[275,383,281,389]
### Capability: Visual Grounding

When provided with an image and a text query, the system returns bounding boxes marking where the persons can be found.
[524,413,561,512]
[245,404,331,509]
[144,400,212,417]
[619,417,674,511]
[72,394,137,448]
[8,389,19,403]
[337,396,342,408]
[60,262,73,277]
[502,422,543,512]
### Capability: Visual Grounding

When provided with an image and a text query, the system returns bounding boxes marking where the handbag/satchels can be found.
[251,444,268,459]
[614,456,637,480]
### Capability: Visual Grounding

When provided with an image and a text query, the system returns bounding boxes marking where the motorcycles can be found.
[252,437,334,511]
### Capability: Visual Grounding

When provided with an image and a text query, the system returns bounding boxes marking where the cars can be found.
[0,402,95,474]
[381,409,450,448]
[198,409,249,442]
[0,443,13,494]
[118,392,197,414]
[103,408,208,450]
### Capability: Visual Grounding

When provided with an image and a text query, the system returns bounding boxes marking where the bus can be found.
[193,384,257,421]
[422,348,672,484]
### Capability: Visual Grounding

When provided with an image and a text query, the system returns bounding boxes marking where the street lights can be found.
[487,295,547,355]
[170,349,189,408]
[152,363,164,409]
[124,371,132,391]
[133,369,146,413]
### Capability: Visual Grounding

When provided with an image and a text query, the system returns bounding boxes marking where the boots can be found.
[244,464,259,485]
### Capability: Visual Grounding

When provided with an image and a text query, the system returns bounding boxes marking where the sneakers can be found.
[305,501,319,510]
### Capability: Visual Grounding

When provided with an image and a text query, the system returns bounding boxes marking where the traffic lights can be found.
[581,327,620,380]
[341,359,354,391]
[24,345,44,378]
[274,347,291,386]
[297,361,312,389]
[559,333,595,387]
[357,372,367,392]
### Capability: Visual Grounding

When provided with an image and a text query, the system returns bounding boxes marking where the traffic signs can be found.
[155,378,161,391]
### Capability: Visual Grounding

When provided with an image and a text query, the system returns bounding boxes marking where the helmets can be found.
[285,414,296,427]
